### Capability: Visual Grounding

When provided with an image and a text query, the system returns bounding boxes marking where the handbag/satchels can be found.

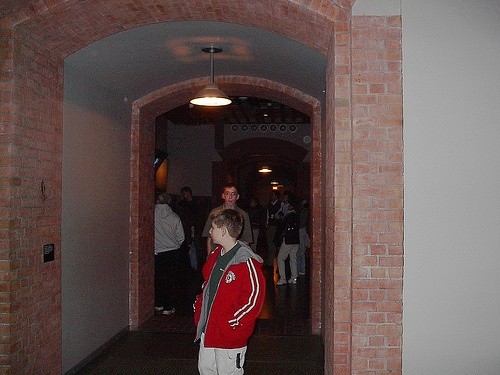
[306,233,311,248]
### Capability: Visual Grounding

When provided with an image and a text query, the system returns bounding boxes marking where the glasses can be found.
[225,192,238,195]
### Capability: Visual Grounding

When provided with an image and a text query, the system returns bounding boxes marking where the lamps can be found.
[190,48,231,107]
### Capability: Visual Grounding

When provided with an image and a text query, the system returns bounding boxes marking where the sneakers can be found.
[277,279,286,285]
[288,279,297,284]
[154,306,163,311]
[163,308,175,315]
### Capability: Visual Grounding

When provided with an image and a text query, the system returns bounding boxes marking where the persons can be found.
[193,209,266,375]
[201,183,254,255]
[155,185,310,315]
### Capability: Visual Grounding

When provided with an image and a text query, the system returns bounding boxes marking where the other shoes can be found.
[298,273,306,275]
